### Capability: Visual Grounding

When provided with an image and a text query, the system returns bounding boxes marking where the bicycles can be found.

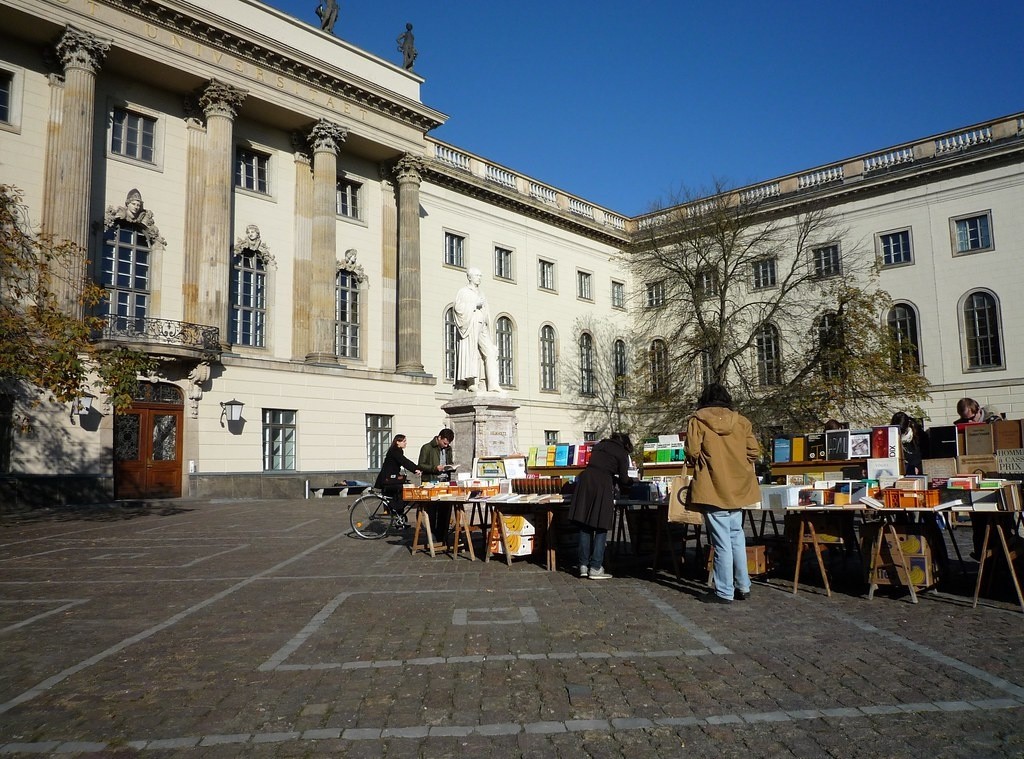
[347,470,429,540]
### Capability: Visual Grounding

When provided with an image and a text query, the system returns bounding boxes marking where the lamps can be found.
[73,389,93,416]
[220,398,245,421]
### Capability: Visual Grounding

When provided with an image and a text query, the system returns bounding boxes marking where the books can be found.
[403,419,1024,510]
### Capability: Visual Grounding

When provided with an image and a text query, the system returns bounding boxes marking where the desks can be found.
[784,506,1023,611]
[406,497,781,590]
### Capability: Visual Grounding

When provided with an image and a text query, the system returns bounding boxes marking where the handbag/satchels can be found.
[383,475,406,484]
[668,458,703,525]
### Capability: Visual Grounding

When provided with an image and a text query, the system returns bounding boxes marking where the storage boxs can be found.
[495,512,537,535]
[861,554,936,588]
[781,514,844,543]
[884,487,942,509]
[402,486,500,501]
[485,528,538,557]
[703,538,776,574]
[859,522,936,555]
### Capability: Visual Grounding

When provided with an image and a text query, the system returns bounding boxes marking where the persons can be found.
[568,432,634,580]
[374,434,422,529]
[953,398,1014,561]
[452,266,510,392]
[685,383,760,604]
[418,428,454,544]
[245,224,261,250]
[345,249,357,271]
[126,189,144,218]
[890,412,939,524]
[334,479,375,494]
[823,420,854,549]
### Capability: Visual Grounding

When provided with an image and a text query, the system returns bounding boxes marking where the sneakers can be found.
[589,565,613,579]
[578,565,589,577]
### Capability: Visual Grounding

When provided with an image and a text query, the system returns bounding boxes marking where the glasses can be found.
[961,413,976,420]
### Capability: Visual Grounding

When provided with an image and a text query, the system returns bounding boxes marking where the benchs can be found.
[311,485,372,498]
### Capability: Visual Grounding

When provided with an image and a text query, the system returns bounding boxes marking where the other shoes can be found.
[698,591,731,604]
[395,523,411,529]
[734,589,751,601]
